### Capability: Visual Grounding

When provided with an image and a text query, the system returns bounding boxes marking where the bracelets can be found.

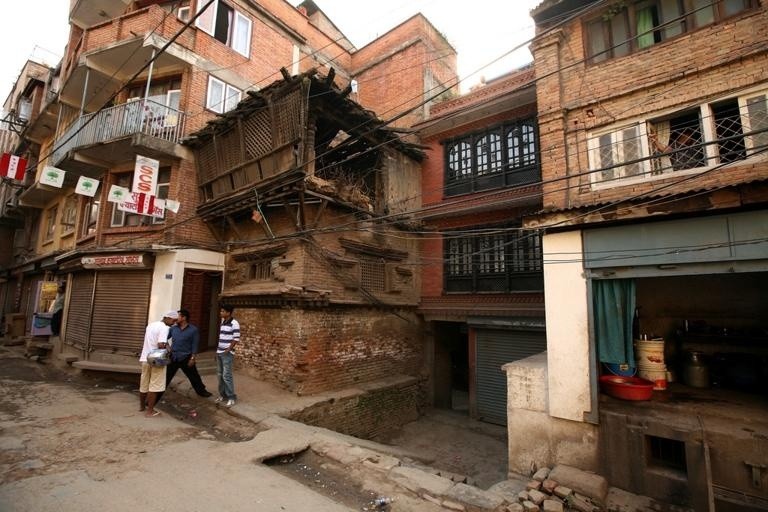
[190,356,195,360]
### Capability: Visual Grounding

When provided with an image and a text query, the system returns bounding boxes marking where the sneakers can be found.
[226,399,235,406]
[197,390,213,398]
[214,396,224,404]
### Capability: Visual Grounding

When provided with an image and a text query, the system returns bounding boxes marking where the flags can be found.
[74,176,101,199]
[37,165,67,190]
[137,192,157,214]
[0,152,28,181]
[106,182,130,205]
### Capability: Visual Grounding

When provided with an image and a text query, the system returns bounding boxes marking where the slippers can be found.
[145,411,161,417]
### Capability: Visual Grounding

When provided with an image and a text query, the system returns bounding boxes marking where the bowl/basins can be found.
[599,375,655,401]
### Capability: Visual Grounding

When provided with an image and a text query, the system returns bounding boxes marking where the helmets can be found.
[147,348,172,367]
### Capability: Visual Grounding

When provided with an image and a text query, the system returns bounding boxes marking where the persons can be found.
[213,304,241,407]
[146,309,211,408]
[138,311,179,418]
[51,286,65,337]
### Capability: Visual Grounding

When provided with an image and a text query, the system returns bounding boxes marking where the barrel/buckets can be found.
[635,341,665,364]
[637,364,669,391]
[11,315,26,336]
